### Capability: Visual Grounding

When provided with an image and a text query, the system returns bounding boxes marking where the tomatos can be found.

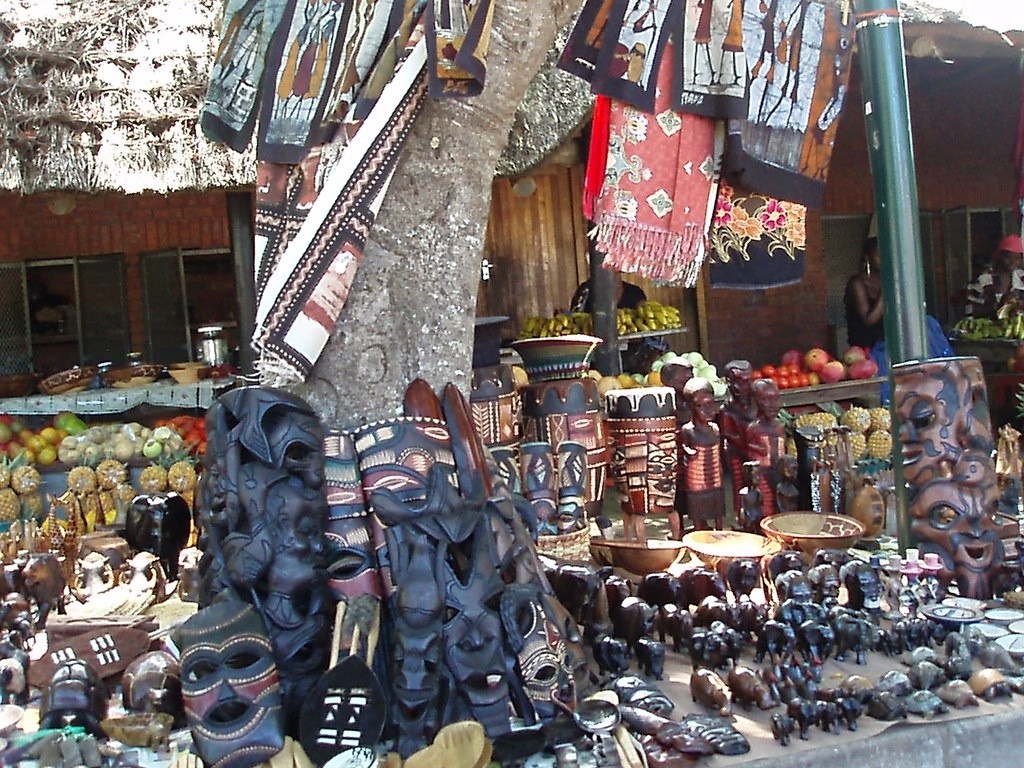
[752,364,808,388]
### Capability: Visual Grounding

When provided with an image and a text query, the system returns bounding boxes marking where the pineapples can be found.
[787,407,893,457]
[0,460,197,536]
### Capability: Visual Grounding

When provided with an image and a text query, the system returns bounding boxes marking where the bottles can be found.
[97,352,148,372]
[196,327,228,367]
[845,457,898,537]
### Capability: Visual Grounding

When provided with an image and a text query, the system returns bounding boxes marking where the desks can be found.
[0,378,235,430]
[616,327,689,375]
[951,339,1019,364]
[774,377,890,413]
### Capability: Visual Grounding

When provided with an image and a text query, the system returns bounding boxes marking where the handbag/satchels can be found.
[871,341,890,405]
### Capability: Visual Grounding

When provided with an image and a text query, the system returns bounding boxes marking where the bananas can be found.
[954,314,1024,343]
[518,300,682,339]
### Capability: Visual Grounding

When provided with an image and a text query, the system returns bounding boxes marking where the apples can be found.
[783,346,877,383]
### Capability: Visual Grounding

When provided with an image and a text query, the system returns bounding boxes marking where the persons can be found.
[739,461,764,534]
[964,233,1024,320]
[842,214,886,352]
[570,277,647,314]
[658,358,714,534]
[879,548,944,620]
[719,359,759,525]
[744,377,788,517]
[774,456,799,514]
[680,377,725,529]
[728,547,883,625]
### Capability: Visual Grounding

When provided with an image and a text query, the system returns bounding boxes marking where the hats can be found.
[999,235,1024,253]
[868,211,878,238]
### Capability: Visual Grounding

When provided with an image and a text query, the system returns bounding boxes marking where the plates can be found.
[921,604,985,622]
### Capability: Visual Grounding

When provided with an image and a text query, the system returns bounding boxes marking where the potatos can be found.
[58,422,152,464]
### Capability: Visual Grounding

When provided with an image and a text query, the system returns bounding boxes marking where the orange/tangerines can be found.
[0,416,63,464]
[155,415,207,453]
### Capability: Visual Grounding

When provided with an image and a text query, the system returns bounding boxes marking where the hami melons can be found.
[513,367,528,384]
[586,370,620,398]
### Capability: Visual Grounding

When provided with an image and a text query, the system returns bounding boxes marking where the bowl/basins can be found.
[588,538,686,575]
[99,365,160,389]
[0,373,38,398]
[759,511,866,553]
[38,366,97,394]
[167,362,206,384]
[682,530,781,574]
[509,335,605,381]
[942,591,1024,659]
[99,712,174,748]
